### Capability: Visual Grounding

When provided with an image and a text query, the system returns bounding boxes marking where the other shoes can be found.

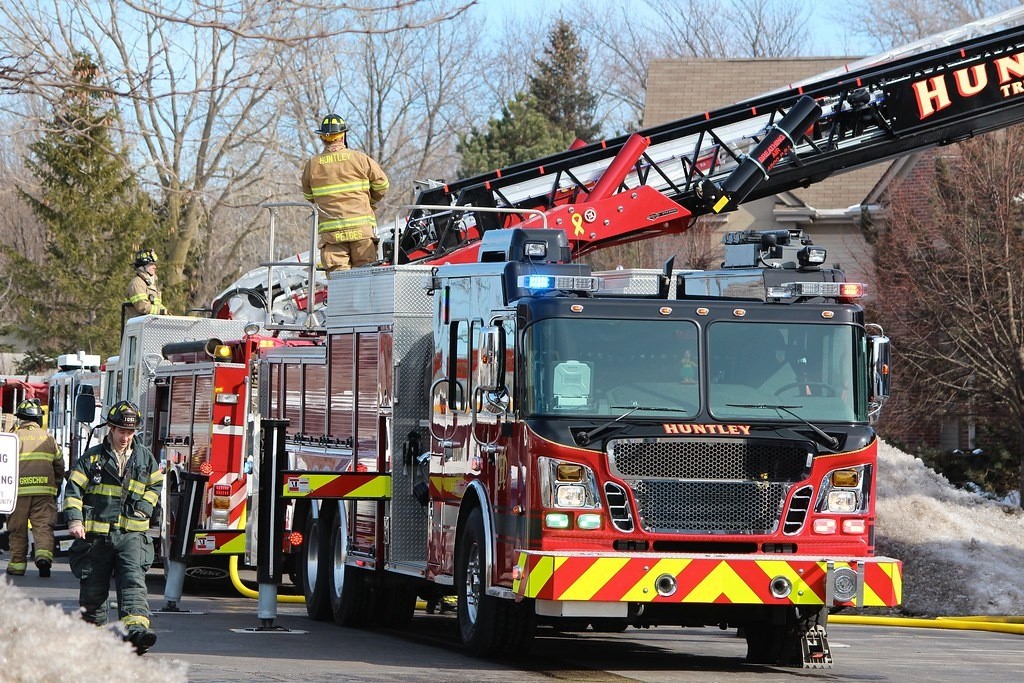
[7,567,26,577]
[37,560,52,577]
[123,625,158,656]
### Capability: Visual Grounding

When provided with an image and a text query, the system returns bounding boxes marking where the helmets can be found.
[13,398,45,420]
[129,249,160,268]
[105,400,145,430]
[314,114,349,136]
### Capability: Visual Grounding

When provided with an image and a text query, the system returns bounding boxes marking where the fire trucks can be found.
[0,8,1024,594]
[242,26,1023,670]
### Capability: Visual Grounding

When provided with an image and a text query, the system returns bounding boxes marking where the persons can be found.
[125,249,173,320]
[302,114,390,279]
[0,398,65,577]
[64,401,163,655]
[728,328,812,396]
